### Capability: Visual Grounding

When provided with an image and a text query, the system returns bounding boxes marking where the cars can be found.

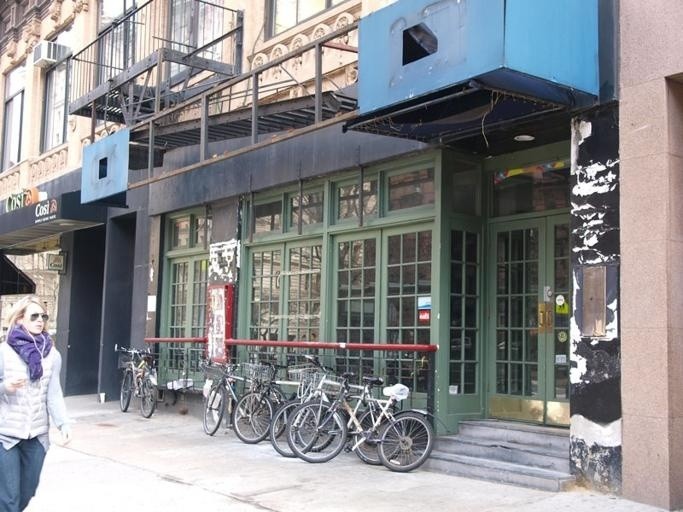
[451,337,472,351]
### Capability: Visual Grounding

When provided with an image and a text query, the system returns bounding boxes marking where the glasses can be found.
[25,310,49,325]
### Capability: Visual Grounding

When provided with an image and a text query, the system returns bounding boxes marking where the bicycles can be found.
[115,344,157,418]
[199,354,434,472]
[250,311,318,365]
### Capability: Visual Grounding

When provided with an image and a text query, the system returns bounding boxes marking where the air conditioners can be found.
[31,40,58,69]
[56,45,73,72]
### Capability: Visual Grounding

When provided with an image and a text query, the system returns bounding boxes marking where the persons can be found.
[0,294,73,512]
